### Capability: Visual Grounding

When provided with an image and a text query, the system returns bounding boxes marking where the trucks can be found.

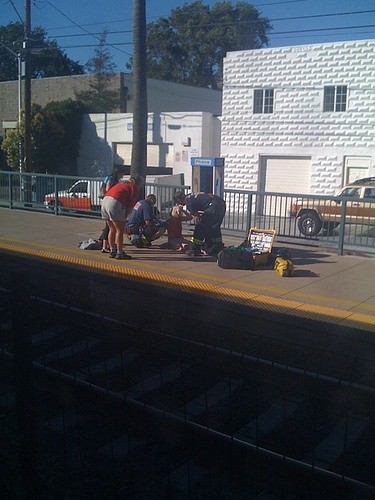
[43,173,184,214]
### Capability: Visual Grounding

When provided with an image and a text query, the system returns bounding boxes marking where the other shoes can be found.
[109,252,117,258]
[115,251,132,260]
[101,248,110,253]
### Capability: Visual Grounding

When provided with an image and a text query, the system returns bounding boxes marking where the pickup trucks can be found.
[292,177,375,236]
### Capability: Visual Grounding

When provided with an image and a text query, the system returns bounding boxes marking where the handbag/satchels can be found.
[217,248,244,269]
[274,257,293,276]
[98,175,110,199]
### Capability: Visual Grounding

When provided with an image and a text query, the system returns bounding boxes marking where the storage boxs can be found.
[248,226,277,266]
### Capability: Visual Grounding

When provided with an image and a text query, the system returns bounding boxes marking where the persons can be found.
[159,203,194,253]
[99,164,123,253]
[125,194,168,248]
[100,177,138,260]
[173,191,226,256]
